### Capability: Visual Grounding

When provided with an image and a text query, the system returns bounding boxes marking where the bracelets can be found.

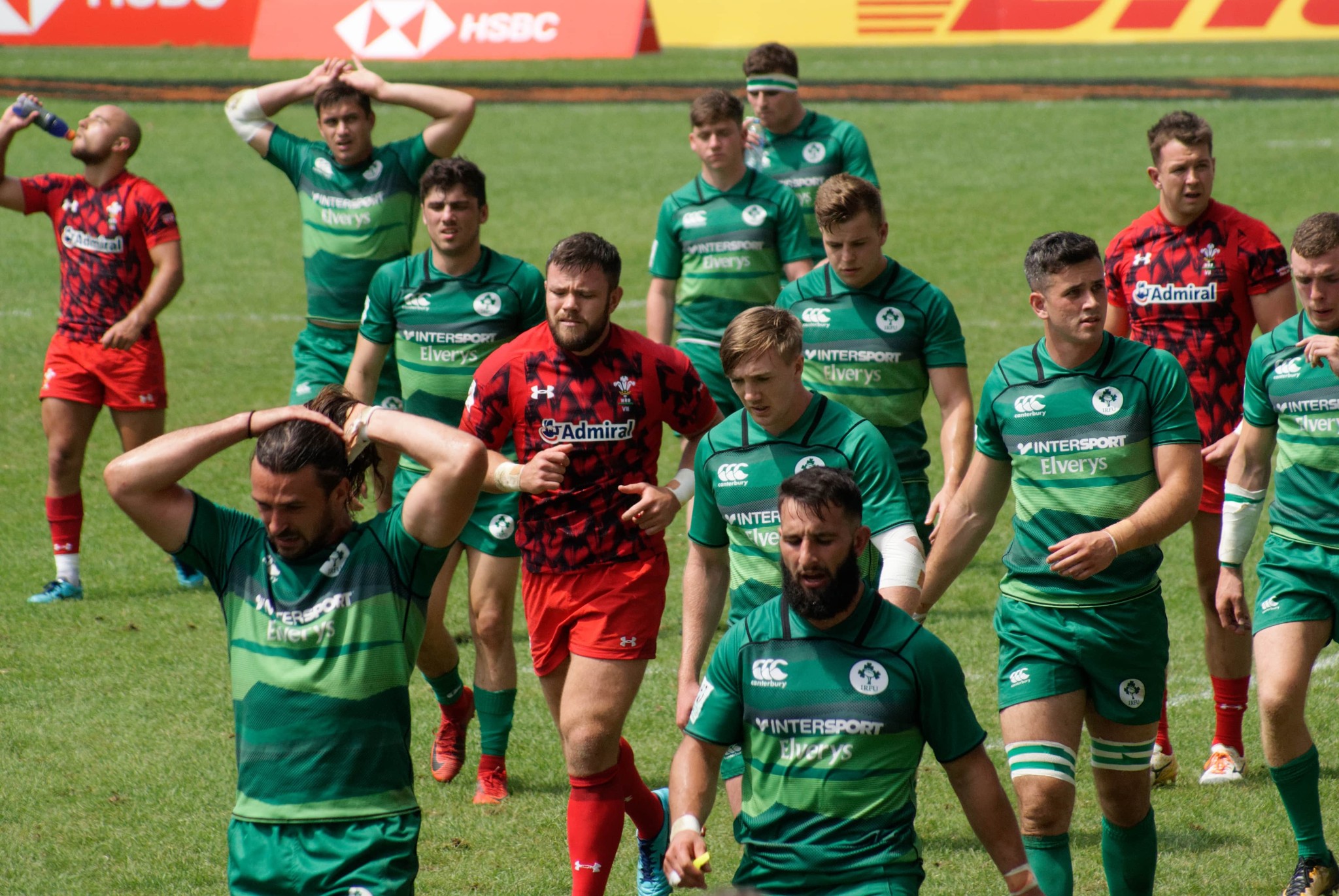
[1003,862,1037,895]
[247,410,255,439]
[362,405,384,441]
[668,815,701,842]
[493,461,529,495]
[664,468,700,508]
[1103,519,1141,557]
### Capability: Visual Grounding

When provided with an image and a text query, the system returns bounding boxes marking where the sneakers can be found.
[430,685,475,783]
[1199,742,1249,786]
[169,553,204,587]
[27,576,84,604]
[1281,849,1339,896]
[1148,746,1179,789]
[473,766,509,804]
[637,787,673,896]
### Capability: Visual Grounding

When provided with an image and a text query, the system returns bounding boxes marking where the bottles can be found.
[12,95,76,140]
[743,118,764,171]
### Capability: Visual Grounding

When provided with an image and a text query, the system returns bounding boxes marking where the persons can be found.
[915,233,1204,896]
[1104,109,1299,789]
[676,305,925,826]
[723,42,881,288]
[224,55,474,512]
[1214,212,1339,896]
[773,172,976,647]
[662,465,1045,896]
[643,88,813,555]
[457,231,726,896]
[340,155,549,804]
[103,385,489,895]
[0,93,205,603]
